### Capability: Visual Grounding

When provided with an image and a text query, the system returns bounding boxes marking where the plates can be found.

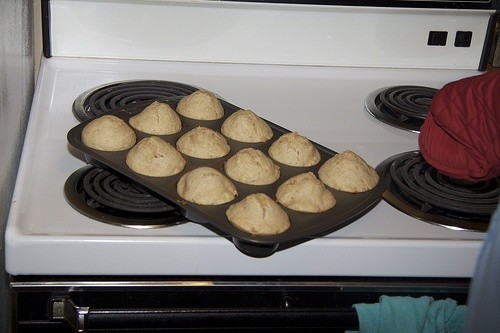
[67,95,386,258]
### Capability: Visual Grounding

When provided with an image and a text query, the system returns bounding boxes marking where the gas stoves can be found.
[5,1,500,276]
